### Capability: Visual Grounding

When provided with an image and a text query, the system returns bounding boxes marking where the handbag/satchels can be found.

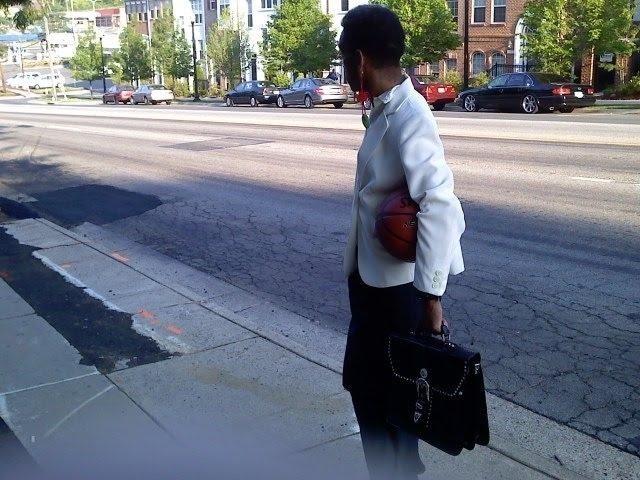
[382,320,491,456]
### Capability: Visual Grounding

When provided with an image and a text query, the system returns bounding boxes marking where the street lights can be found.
[98,34,106,92]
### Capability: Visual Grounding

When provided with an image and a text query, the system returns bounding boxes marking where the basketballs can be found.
[376,188,420,262]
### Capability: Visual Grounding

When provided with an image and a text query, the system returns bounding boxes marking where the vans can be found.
[20,48,41,61]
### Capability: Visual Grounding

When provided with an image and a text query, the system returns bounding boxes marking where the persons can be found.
[336,5,468,480]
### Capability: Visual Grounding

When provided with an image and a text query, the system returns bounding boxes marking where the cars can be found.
[103,84,174,105]
[5,72,65,90]
